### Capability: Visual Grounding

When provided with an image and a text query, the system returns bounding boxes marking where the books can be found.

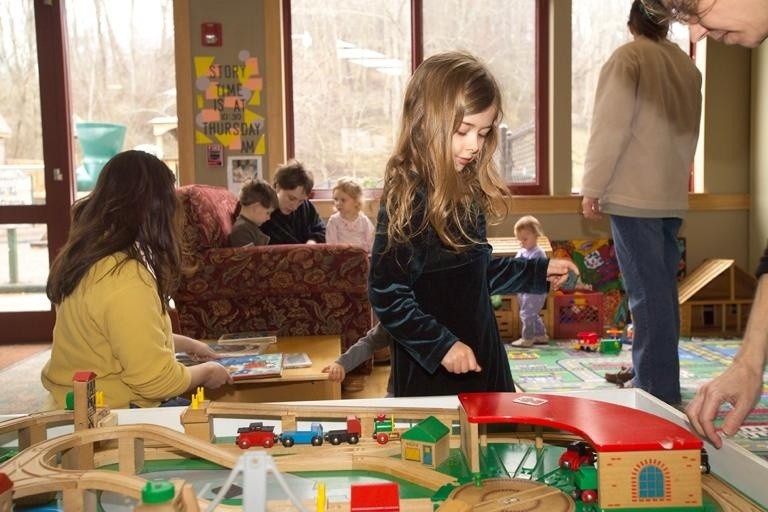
[175,329,312,381]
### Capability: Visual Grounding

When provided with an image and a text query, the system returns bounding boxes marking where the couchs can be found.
[170,184,374,377]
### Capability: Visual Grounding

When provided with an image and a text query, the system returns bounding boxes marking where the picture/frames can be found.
[226,155,263,201]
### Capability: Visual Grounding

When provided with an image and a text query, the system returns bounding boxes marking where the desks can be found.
[177,334,342,402]
[0,386,766,512]
[488,235,554,258]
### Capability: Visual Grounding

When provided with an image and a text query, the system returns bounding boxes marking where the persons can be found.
[644,0,768,450]
[261,160,327,246]
[325,178,376,257]
[228,178,278,244]
[511,216,549,347]
[322,322,397,398]
[367,51,579,396]
[580,0,701,403]
[40,150,232,410]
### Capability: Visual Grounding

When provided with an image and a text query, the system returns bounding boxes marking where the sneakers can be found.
[511,337,534,348]
[534,336,549,344]
[605,367,633,382]
